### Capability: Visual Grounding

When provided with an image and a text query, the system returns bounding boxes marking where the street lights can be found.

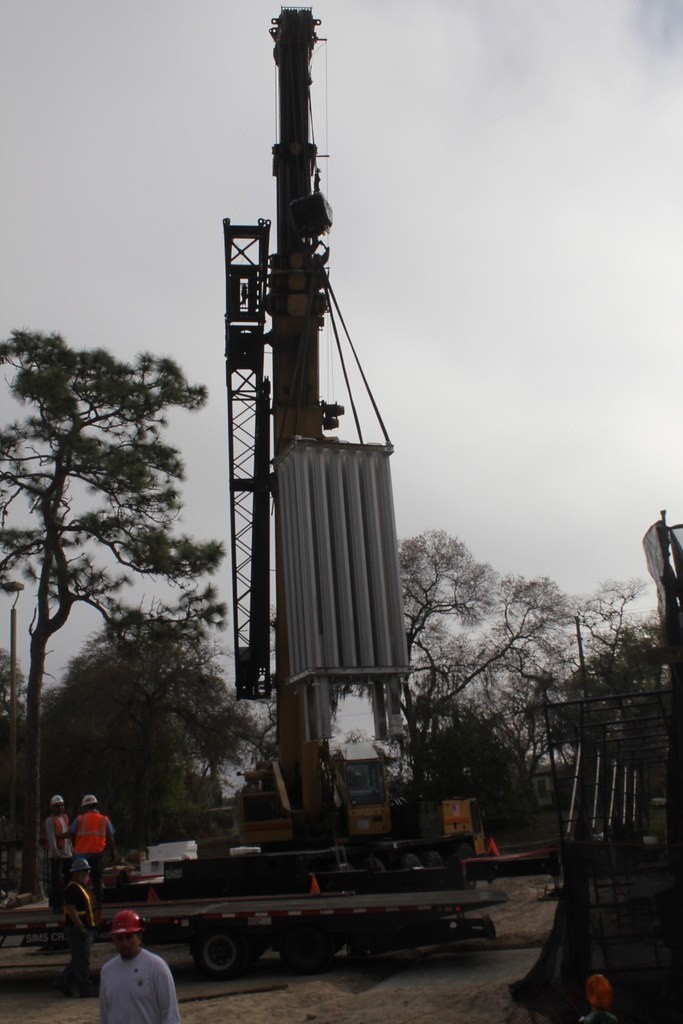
[2,581,25,890]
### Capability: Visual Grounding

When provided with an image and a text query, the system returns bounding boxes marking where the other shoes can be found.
[52,980,74,997]
[80,989,100,997]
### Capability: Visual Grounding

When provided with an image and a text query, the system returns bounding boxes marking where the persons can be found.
[350,771,368,789]
[45,794,74,914]
[54,858,100,998]
[100,909,182,1024]
[577,973,618,1024]
[55,794,116,908]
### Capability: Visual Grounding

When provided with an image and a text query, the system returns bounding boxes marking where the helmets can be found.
[50,795,64,806]
[69,858,91,872]
[80,795,97,807]
[108,909,148,934]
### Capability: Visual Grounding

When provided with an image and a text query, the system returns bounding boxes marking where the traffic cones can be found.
[486,836,502,859]
[309,873,321,895]
[145,886,161,902]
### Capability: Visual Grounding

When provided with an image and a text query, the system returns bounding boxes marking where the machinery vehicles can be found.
[93,7,498,894]
